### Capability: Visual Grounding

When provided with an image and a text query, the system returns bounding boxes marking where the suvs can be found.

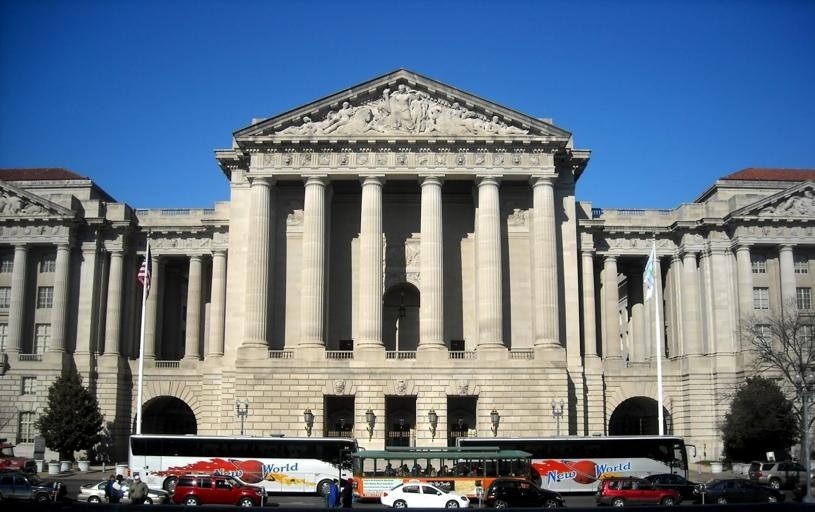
[483,478,566,509]
[595,474,683,507]
[0,471,67,502]
[171,475,269,507]
[749,460,815,490]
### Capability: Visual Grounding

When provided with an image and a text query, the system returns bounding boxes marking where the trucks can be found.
[0,437,36,475]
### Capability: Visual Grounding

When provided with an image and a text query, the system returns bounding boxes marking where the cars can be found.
[694,476,786,504]
[77,480,169,504]
[645,473,706,500]
[380,481,471,510]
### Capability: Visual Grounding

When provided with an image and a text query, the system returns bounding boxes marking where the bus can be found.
[456,435,697,494]
[349,446,534,501]
[127,432,365,495]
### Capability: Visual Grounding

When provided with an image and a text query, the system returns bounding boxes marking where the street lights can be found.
[793,368,815,502]
[551,398,565,436]
[397,415,406,446]
[235,397,249,435]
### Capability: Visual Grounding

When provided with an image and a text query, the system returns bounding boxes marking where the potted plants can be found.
[709,461,751,477]
[34,456,91,476]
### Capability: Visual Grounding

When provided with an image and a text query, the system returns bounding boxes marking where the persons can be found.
[329,478,339,508]
[105,474,116,503]
[383,460,481,477]
[128,475,148,503]
[340,479,352,508]
[107,473,123,503]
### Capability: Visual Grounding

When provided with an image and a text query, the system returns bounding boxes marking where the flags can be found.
[643,249,655,302]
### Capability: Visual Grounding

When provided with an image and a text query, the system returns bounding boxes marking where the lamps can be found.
[428,407,437,434]
[304,408,313,436]
[491,408,500,436]
[366,406,375,436]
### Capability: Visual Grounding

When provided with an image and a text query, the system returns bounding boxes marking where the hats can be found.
[134,475,140,480]
